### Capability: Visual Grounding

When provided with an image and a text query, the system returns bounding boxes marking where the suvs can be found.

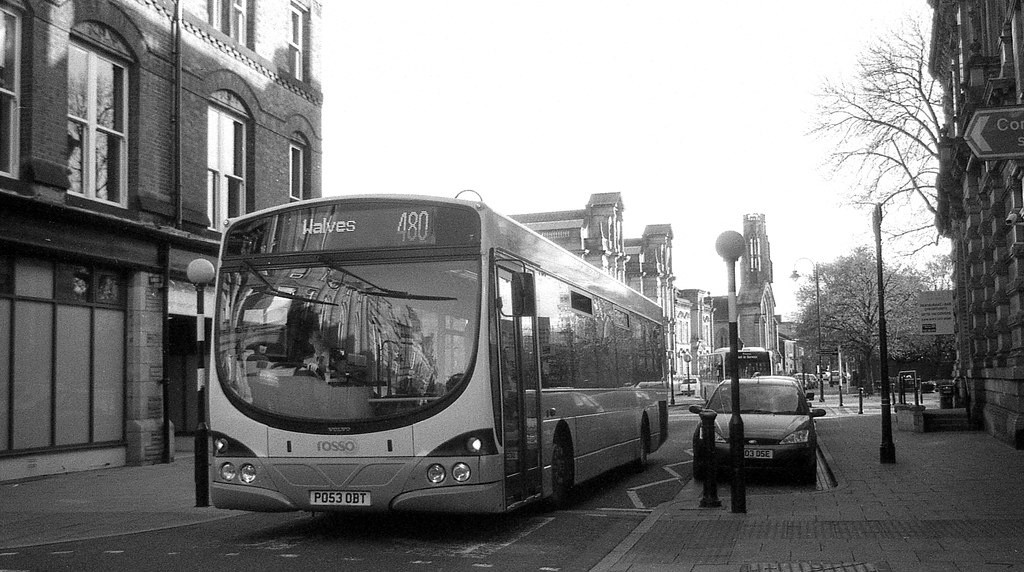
[680,379,699,395]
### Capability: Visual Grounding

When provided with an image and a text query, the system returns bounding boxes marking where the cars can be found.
[792,371,856,389]
[689,378,826,488]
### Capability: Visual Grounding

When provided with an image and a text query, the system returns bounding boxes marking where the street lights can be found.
[684,354,693,398]
[714,231,747,512]
[186,258,215,507]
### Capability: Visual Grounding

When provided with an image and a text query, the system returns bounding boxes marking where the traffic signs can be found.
[963,103,1024,160]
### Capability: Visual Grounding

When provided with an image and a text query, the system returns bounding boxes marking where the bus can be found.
[698,346,773,407]
[207,189,668,513]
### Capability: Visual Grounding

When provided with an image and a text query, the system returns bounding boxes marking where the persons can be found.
[297,327,347,377]
